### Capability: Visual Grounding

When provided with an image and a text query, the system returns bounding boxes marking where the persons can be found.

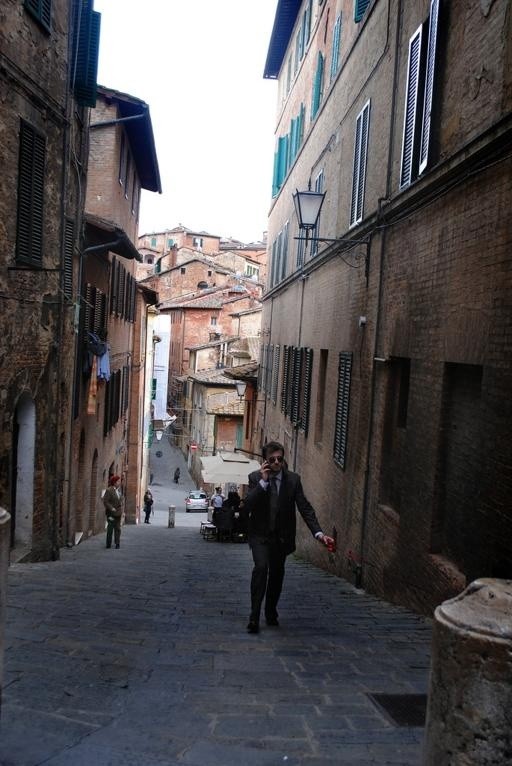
[247,441,334,633]
[103,476,122,549]
[211,486,249,543]
[174,468,180,483]
[144,487,153,523]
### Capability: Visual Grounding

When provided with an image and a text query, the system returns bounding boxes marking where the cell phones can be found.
[262,458,271,473]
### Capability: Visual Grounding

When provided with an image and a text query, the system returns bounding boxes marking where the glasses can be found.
[269,457,283,463]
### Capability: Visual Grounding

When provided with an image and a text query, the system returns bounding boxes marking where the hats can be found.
[110,476,120,485]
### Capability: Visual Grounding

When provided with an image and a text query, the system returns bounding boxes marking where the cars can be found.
[184,490,209,512]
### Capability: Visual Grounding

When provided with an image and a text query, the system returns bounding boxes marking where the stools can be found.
[200,520,233,542]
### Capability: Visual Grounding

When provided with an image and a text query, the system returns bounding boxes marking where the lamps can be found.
[292,179,372,287]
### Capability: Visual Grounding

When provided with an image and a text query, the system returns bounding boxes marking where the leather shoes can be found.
[246,621,259,634]
[106,544,120,549]
[266,618,279,626]
